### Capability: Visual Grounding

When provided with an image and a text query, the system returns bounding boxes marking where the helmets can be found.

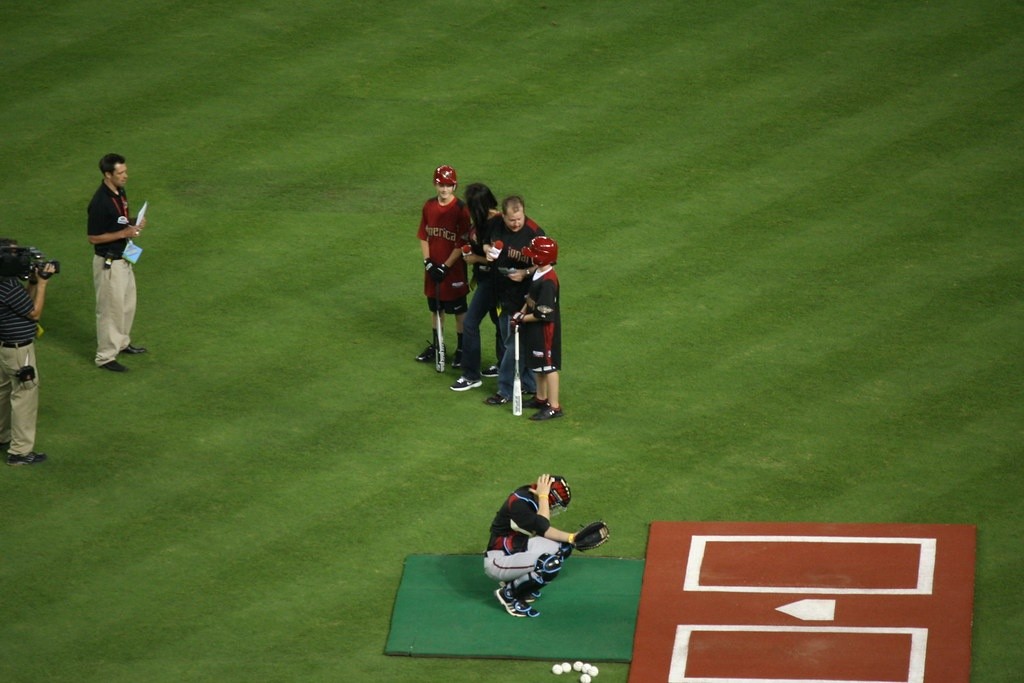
[546,476,571,516]
[521,236,558,266]
[433,166,458,192]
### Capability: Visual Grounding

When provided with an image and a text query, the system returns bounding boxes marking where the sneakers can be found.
[480,363,501,378]
[495,587,526,618]
[8,451,47,466]
[530,404,564,420]
[523,595,536,604]
[487,395,508,404]
[522,397,547,409]
[450,376,482,392]
[416,339,446,362]
[0,442,11,451]
[452,351,462,368]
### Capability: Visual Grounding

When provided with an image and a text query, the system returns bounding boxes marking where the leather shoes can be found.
[102,361,125,371]
[123,345,146,354]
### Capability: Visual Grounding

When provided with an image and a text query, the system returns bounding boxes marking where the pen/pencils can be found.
[127,225,142,231]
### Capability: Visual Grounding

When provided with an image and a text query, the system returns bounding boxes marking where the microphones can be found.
[461,245,472,256]
[486,240,504,269]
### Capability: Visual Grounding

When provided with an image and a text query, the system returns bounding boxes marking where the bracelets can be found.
[525,267,531,275]
[538,494,549,500]
[568,533,575,544]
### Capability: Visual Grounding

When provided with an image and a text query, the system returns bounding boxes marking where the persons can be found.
[482,473,611,617]
[414,165,472,367]
[85,152,147,374]
[508,237,564,420]
[1,237,58,466]
[479,196,545,404]
[450,182,507,392]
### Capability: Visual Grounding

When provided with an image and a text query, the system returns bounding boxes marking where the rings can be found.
[135,231,140,235]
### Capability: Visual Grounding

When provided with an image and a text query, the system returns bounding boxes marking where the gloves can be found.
[510,311,525,329]
[440,264,450,276]
[485,247,502,262]
[423,257,443,282]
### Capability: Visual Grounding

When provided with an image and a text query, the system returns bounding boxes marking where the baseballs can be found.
[551,661,599,683]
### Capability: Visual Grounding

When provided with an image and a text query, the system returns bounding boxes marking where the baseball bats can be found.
[435,282,446,373]
[512,324,524,416]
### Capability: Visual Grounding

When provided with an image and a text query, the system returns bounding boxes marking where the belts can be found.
[0,339,33,348]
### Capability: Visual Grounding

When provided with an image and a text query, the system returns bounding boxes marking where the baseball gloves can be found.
[572,518,611,553]
